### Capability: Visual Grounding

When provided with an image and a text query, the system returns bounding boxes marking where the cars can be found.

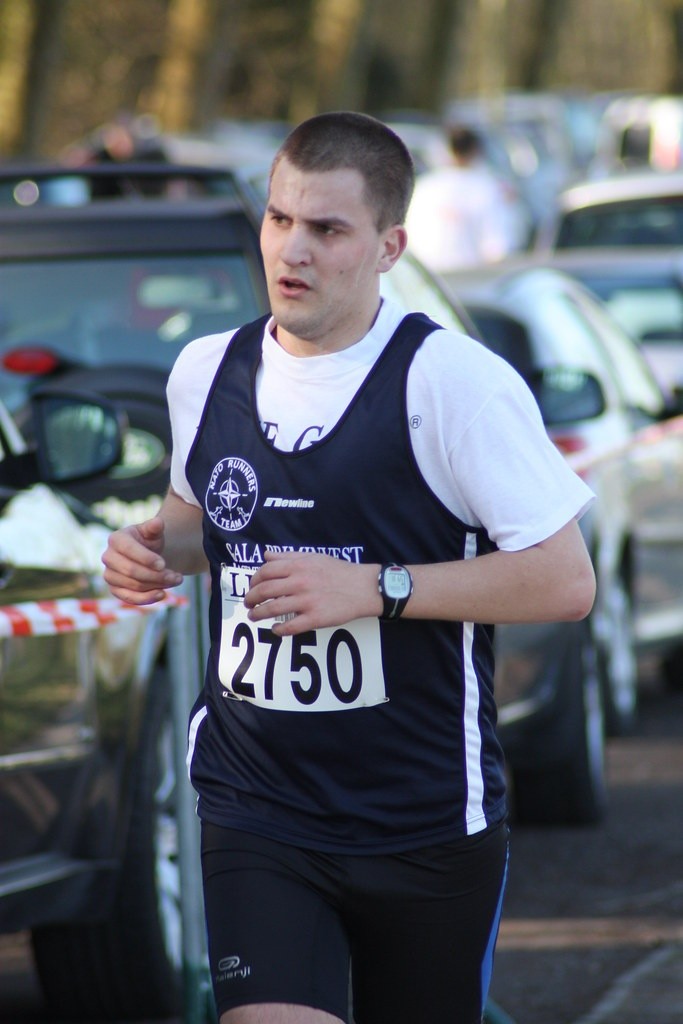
[0,89,681,1024]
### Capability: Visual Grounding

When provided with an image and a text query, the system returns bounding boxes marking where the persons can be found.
[405,125,516,275]
[102,112,598,1024]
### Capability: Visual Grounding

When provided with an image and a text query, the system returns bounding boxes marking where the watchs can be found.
[377,560,412,619]
